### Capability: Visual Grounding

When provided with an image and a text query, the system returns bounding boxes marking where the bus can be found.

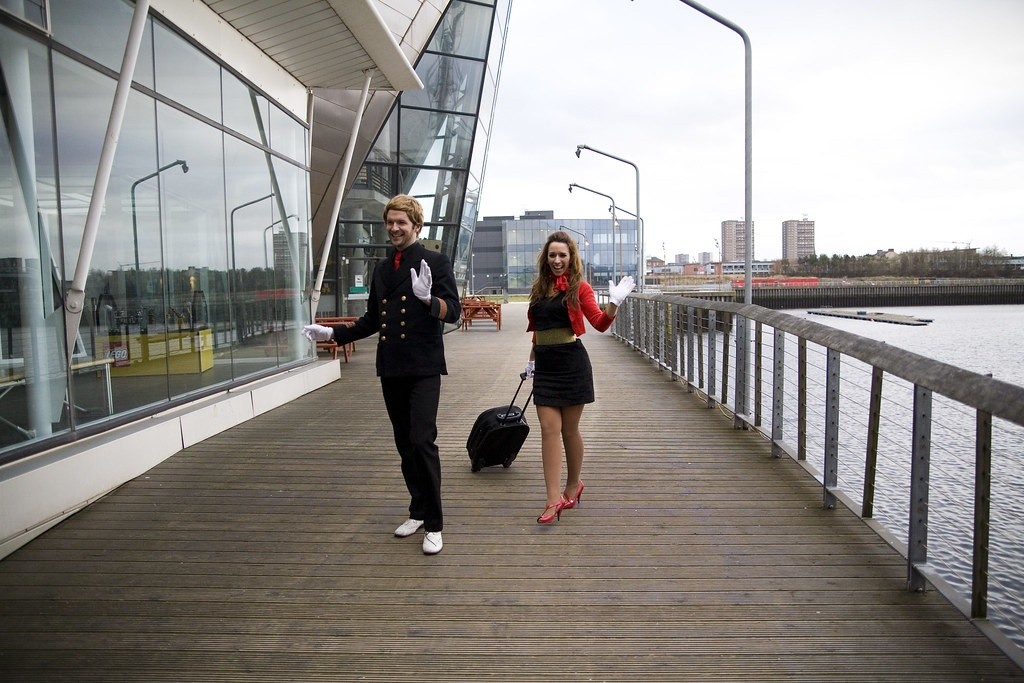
[915,278,937,284]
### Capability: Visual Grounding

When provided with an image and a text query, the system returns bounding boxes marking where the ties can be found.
[394,251,402,271]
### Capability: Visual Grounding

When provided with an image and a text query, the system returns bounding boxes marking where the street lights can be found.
[608,205,644,294]
[559,225,587,282]
[569,183,616,288]
[715,238,720,291]
[131,160,189,306]
[576,145,640,293]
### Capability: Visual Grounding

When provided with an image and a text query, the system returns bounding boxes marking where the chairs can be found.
[460,296,502,331]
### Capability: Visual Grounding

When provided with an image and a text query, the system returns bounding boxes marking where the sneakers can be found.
[394,519,424,536]
[423,531,444,553]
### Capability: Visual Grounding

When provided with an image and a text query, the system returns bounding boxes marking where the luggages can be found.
[466,371,535,472]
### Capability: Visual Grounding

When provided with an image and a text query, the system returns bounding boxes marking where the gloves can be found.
[608,276,636,307]
[300,325,333,342]
[524,360,535,379]
[410,259,433,304]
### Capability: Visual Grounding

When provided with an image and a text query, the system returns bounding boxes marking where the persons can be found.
[301,194,462,555]
[525,231,637,523]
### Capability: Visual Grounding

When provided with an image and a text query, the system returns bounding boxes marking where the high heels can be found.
[561,481,584,509]
[536,496,565,524]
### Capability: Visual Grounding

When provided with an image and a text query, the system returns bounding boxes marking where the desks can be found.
[316,315,362,362]
[0,355,115,457]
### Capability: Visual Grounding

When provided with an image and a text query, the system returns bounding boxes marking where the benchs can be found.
[317,344,350,363]
[461,309,499,332]
